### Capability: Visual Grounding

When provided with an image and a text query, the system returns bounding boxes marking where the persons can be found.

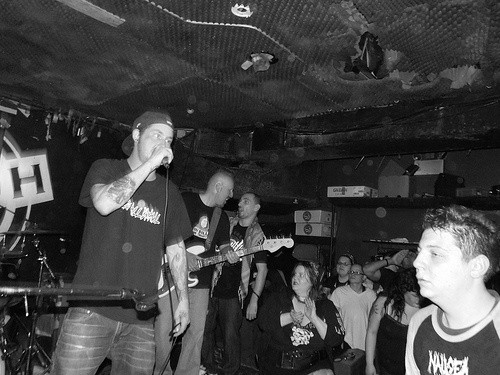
[321,249,433,375]
[404,203,500,375]
[365,266,433,375]
[256,261,345,375]
[199,192,269,375]
[154,167,243,375]
[49,108,194,375]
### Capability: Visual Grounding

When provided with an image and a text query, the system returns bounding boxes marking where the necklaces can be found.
[295,295,305,305]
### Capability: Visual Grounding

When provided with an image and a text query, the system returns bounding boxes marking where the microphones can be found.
[162,156,169,168]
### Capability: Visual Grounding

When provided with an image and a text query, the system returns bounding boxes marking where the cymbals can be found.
[0,229,67,235]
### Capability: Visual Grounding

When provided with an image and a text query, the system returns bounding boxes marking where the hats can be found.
[122,111,174,156]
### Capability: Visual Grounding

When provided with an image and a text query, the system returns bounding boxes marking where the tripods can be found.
[15,233,53,375]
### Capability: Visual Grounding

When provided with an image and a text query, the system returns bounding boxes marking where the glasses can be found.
[336,261,351,266]
[347,270,366,275]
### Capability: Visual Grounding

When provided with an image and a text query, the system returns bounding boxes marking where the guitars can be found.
[157,235,294,299]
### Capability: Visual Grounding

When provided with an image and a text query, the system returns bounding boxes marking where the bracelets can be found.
[384,258,389,266]
[252,289,260,298]
